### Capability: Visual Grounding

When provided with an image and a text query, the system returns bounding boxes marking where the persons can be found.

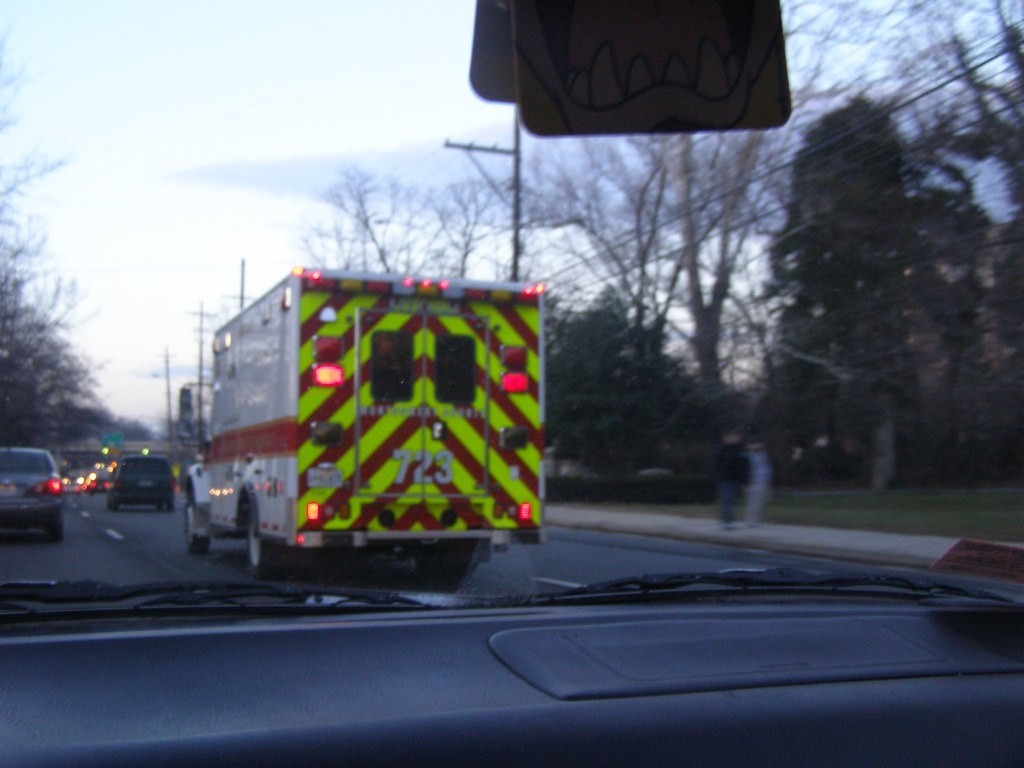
[742,436,774,527]
[712,428,750,530]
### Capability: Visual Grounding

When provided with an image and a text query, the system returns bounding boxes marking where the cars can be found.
[63,469,88,494]
[107,452,175,512]
[0,445,66,541]
[85,468,113,496]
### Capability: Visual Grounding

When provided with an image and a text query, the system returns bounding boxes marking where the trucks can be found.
[182,267,548,590]
